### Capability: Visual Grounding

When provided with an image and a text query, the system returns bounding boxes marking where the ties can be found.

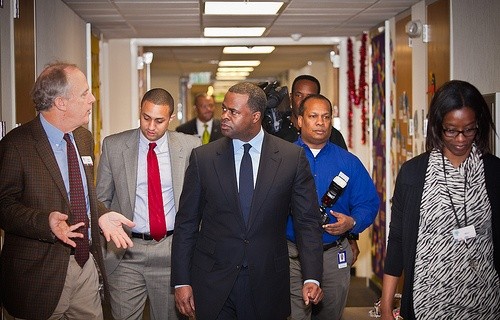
[62,133,89,269]
[146,142,167,242]
[239,145,255,229]
[203,123,209,146]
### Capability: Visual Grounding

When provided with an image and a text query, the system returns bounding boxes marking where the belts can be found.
[323,235,347,251]
[131,230,174,241]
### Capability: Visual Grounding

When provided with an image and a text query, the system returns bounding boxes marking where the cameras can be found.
[319,171,349,226]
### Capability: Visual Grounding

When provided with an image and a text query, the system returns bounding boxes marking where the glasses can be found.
[441,123,478,137]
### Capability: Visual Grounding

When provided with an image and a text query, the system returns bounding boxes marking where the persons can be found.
[175,93,225,145]
[96,88,203,320]
[274,75,347,150]
[0,62,135,320]
[170,82,324,320]
[287,94,381,320]
[380,80,500,320]
